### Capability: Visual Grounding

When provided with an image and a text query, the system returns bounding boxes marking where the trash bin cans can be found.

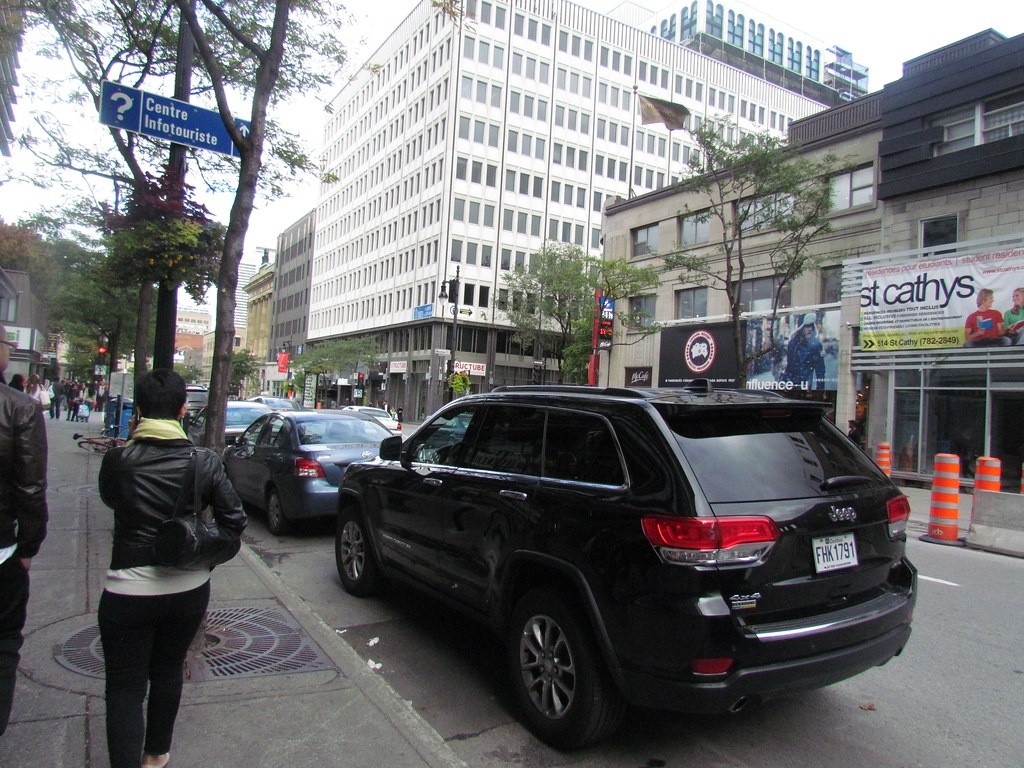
[106,397,134,438]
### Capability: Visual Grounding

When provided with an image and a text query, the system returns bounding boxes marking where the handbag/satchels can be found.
[155,448,241,564]
[78,405,90,418]
[48,386,54,398]
[40,384,51,409]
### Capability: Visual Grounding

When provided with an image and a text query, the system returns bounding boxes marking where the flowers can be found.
[447,372,471,393]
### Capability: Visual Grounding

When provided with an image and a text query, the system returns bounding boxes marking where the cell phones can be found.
[134,413,140,426]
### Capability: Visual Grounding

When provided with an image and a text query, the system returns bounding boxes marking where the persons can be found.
[390,407,396,420]
[963,289,1013,348]
[50,377,105,423]
[368,401,375,407]
[98,369,248,768]
[1003,287,1024,345]
[0,323,49,735]
[381,400,390,414]
[330,399,336,409]
[782,313,826,390]
[398,405,403,418]
[8,374,46,401]
[848,420,860,445]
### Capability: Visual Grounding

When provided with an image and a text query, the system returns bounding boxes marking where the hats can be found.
[790,313,819,339]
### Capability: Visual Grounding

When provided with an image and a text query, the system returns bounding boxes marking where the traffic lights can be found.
[357,373,365,389]
[97,341,107,364]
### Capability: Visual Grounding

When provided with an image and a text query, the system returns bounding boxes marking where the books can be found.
[977,316,992,330]
[1013,320,1024,331]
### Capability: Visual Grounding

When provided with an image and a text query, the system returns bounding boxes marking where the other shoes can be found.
[141,752,170,768]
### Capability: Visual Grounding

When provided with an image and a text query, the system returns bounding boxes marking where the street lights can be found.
[438,265,461,403]
[281,339,292,391]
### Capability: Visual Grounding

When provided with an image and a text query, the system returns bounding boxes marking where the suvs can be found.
[334,378,918,753]
[184,384,210,417]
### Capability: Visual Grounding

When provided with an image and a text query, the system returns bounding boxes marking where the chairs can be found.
[329,422,352,439]
[298,425,306,441]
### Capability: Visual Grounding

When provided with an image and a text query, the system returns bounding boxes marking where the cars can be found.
[222,410,394,537]
[342,405,404,440]
[250,397,303,412]
[190,401,281,448]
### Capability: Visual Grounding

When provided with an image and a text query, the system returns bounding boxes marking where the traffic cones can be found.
[1021,463,1024,494]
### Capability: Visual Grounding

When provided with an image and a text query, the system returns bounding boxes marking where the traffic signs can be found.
[97,78,252,158]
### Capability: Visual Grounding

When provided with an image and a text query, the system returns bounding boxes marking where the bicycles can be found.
[73,425,130,454]
